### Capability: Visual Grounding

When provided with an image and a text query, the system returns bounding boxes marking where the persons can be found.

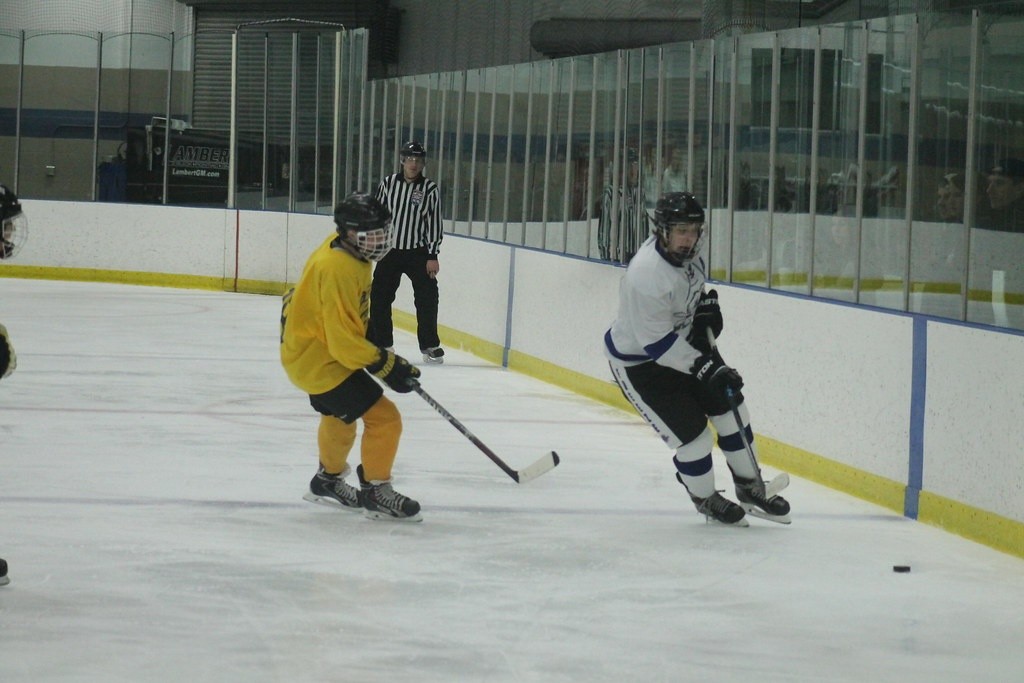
[595,135,1024,272]
[365,142,443,358]
[280,191,420,518]
[0,186,19,587]
[602,191,791,526]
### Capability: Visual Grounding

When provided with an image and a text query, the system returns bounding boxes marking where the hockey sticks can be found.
[704,324,791,501]
[406,377,560,483]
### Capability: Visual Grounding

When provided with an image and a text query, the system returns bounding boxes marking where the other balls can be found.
[893,565,912,574]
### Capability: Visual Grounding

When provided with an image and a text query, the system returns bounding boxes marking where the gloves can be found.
[365,346,421,393]
[690,346,744,416]
[691,289,723,349]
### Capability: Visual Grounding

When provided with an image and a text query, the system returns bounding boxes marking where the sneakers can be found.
[727,461,793,525]
[357,464,424,525]
[301,458,366,513]
[419,346,445,365]
[676,471,750,529]
[1,557,9,586]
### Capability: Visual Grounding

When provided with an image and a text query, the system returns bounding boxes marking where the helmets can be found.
[334,192,396,263]
[398,140,428,157]
[0,183,30,261]
[652,189,710,265]
